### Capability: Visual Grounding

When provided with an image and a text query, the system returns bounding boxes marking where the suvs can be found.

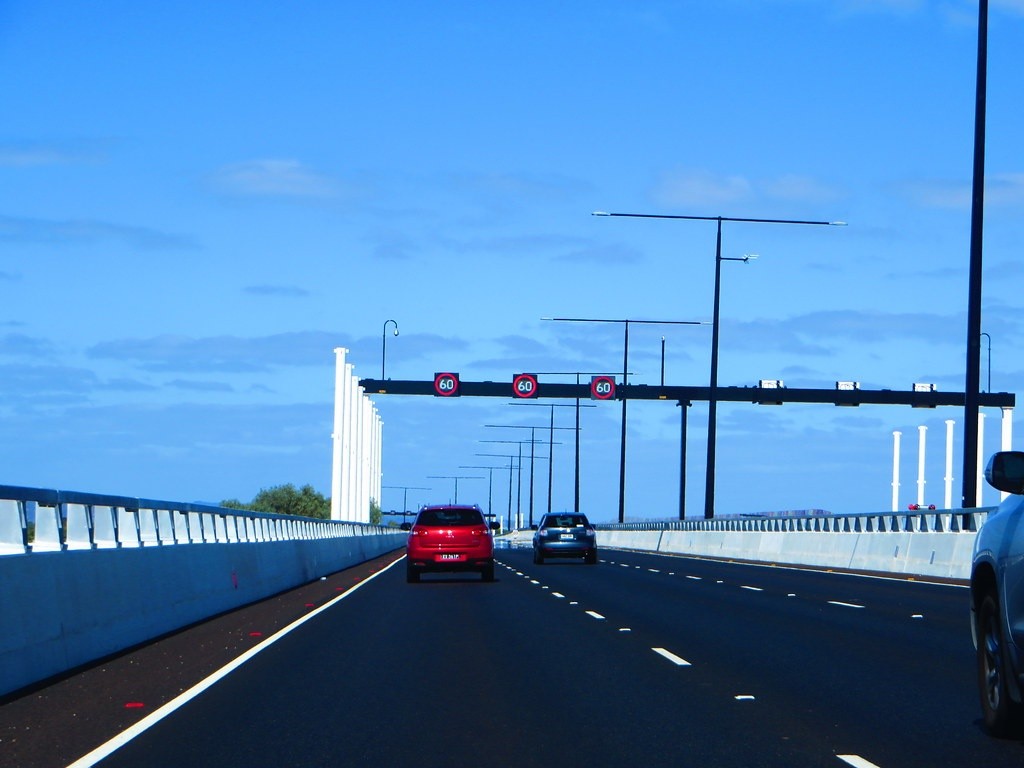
[400,503,500,584]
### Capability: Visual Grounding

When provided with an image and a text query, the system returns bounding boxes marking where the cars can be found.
[968,446,1024,740]
[530,512,598,565]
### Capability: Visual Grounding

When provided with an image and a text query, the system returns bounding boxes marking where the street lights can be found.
[541,317,713,523]
[593,211,848,516]
[522,372,641,509]
[460,465,522,527]
[484,423,583,529]
[475,452,549,532]
[503,400,599,514]
[382,482,435,529]
[427,475,486,504]
[479,439,564,532]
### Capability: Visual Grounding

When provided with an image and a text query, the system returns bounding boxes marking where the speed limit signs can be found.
[434,371,460,397]
[512,374,538,399]
[590,375,617,400]
